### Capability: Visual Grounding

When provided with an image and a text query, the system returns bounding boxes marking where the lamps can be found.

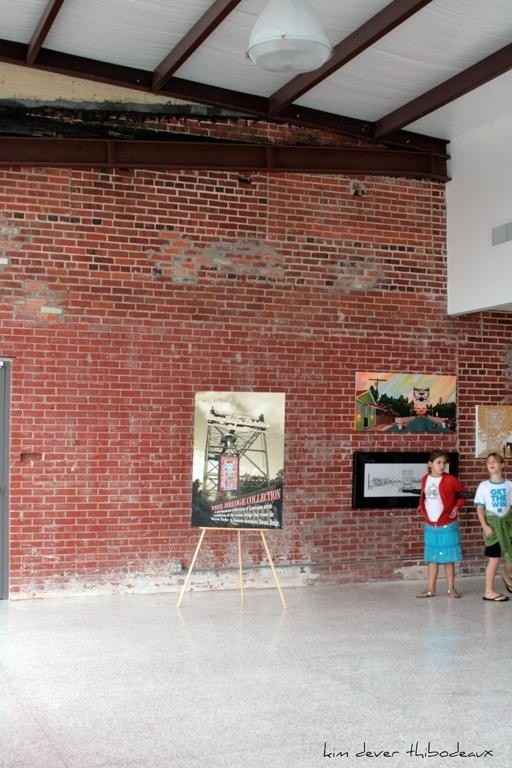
[243,0,333,75]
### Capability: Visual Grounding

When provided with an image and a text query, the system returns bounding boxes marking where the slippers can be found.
[416,591,435,597]
[448,589,460,598]
[483,593,509,601]
[502,577,512,593]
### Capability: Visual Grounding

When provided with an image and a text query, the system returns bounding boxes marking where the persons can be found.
[414,450,466,598]
[474,452,512,601]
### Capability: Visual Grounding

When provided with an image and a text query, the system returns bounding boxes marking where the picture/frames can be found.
[351,450,458,508]
